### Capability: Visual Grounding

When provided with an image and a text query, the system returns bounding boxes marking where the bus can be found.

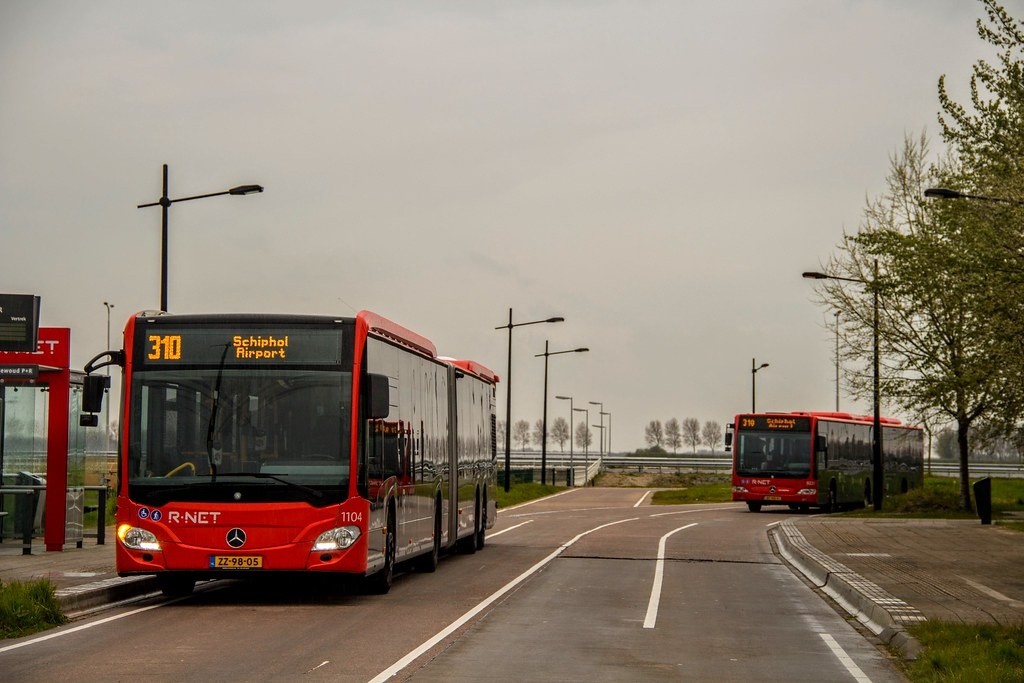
[731,411,924,514]
[79,310,500,604]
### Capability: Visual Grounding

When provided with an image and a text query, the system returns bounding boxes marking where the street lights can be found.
[752,358,769,413]
[802,259,883,514]
[592,425,606,456]
[104,302,115,451]
[535,340,589,484]
[573,408,588,486]
[555,396,574,485]
[599,412,611,456]
[589,402,603,476]
[137,164,264,313]
[495,308,564,492]
